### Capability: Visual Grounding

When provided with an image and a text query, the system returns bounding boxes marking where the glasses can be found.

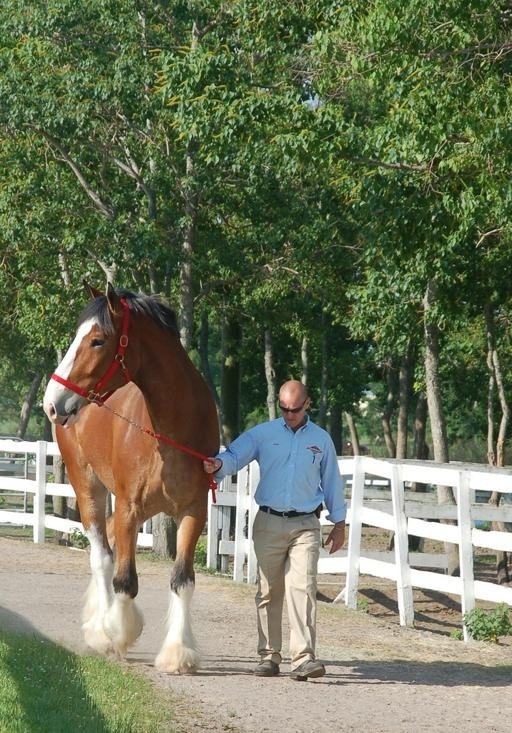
[278,398,307,414]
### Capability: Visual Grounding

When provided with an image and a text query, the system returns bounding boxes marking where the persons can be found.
[204,379,347,681]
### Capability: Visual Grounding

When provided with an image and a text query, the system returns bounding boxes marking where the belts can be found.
[259,505,317,520]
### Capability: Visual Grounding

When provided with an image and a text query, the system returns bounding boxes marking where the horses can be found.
[38,278,222,678]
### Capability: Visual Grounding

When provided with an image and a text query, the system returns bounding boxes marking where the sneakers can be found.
[254,659,279,677]
[290,659,326,681]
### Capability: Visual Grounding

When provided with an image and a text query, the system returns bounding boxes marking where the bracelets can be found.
[334,526,344,531]
